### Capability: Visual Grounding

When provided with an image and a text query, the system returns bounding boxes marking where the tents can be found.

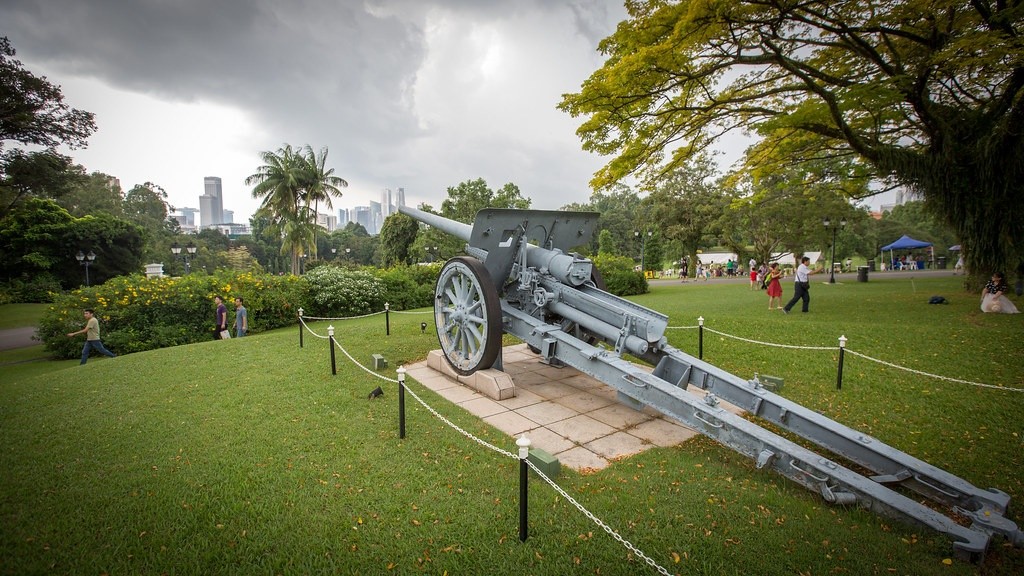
[882,235,935,271]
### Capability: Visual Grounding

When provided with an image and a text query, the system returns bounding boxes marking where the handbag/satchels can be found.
[220,330,231,340]
[800,281,810,290]
[764,274,772,286]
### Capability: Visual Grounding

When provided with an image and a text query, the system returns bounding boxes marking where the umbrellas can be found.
[948,244,962,250]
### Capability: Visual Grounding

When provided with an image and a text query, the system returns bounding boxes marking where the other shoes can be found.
[777,307,783,310]
[768,307,776,311]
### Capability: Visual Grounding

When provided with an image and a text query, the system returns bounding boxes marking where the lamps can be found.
[421,322,427,333]
[368,386,384,398]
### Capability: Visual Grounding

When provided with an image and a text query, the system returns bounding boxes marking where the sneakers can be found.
[782,307,789,315]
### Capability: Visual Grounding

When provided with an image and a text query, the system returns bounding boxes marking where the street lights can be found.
[76,249,97,287]
[822,213,847,284]
[424,244,438,263]
[634,226,653,273]
[172,239,197,275]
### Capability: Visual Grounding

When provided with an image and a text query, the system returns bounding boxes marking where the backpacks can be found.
[929,296,949,305]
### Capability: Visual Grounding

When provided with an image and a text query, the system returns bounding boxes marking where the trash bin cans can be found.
[857,265,871,283]
[937,256,946,270]
[867,259,876,271]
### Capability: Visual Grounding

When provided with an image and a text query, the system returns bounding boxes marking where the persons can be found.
[214,296,228,340]
[782,257,823,314]
[67,309,117,366]
[767,263,783,310]
[980,271,1008,302]
[660,251,966,291]
[232,297,248,338]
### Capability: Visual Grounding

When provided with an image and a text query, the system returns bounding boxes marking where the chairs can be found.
[910,261,918,270]
[899,261,908,271]
